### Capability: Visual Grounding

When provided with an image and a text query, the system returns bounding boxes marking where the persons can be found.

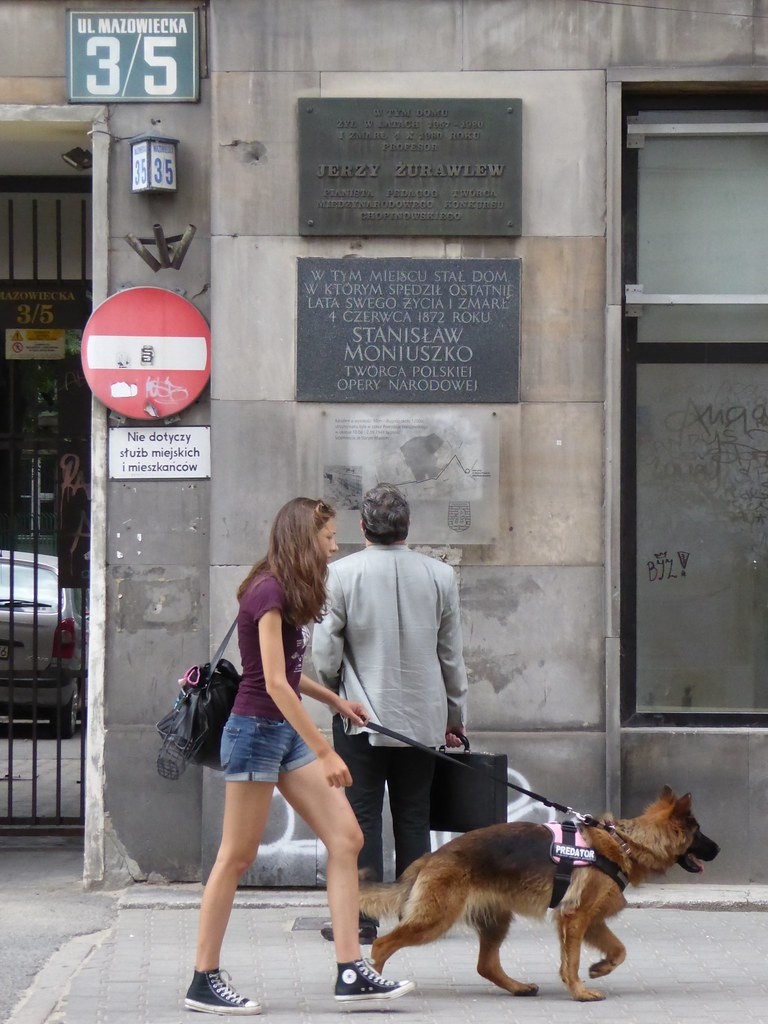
[185,496,415,1016]
[312,481,468,947]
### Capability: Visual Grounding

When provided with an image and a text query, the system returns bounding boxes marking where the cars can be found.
[0,550,91,739]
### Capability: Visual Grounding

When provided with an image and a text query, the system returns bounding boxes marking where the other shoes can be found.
[320,920,377,946]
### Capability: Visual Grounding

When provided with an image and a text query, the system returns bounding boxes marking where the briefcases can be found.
[429,730,508,833]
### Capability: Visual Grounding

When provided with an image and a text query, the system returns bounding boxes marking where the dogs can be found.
[358,785,723,1002]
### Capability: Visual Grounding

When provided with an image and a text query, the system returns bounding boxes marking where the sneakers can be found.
[334,956,416,1002]
[184,967,262,1015]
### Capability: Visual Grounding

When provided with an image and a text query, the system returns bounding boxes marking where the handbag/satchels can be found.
[157,655,242,781]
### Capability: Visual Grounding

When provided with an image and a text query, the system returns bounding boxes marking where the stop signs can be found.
[79,286,211,420]
